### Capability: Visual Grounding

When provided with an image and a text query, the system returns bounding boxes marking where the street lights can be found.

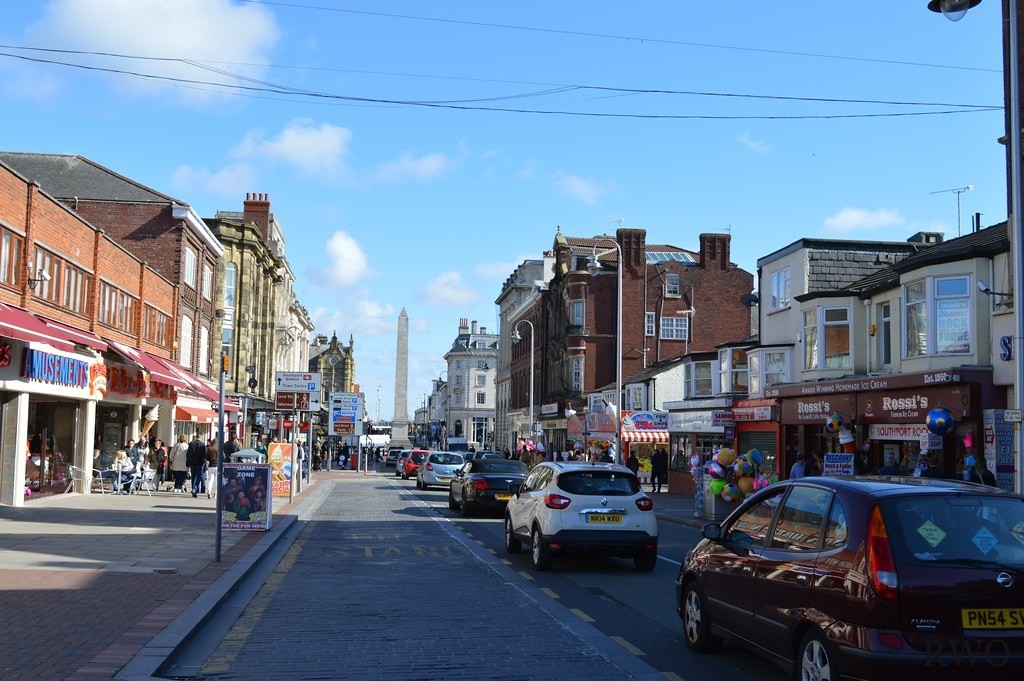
[512,319,534,437]
[438,370,451,437]
[587,238,624,465]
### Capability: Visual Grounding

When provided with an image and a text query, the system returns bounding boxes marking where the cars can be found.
[675,471,1023,680]
[504,461,659,573]
[448,460,529,518]
[417,451,465,491]
[465,450,506,459]
[385,449,430,479]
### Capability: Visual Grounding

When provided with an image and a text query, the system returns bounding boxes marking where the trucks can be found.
[446,436,468,452]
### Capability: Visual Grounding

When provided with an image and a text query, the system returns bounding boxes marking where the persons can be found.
[556,448,624,465]
[627,450,639,477]
[650,447,668,492]
[469,443,531,467]
[93,432,381,498]
[789,447,997,486]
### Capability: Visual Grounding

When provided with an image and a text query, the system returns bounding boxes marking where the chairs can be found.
[61,462,157,496]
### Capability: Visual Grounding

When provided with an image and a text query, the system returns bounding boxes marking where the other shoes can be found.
[173,489,182,493]
[111,491,119,494]
[122,490,131,495]
[657,491,660,493]
[191,489,197,497]
[652,489,656,492]
[207,493,212,498]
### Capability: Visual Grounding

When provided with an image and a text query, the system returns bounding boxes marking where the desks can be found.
[100,468,136,495]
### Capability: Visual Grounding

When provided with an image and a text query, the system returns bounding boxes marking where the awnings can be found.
[103,336,241,412]
[620,432,669,442]
[0,301,109,352]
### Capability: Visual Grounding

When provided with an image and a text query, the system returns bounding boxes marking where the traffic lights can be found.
[366,423,372,435]
